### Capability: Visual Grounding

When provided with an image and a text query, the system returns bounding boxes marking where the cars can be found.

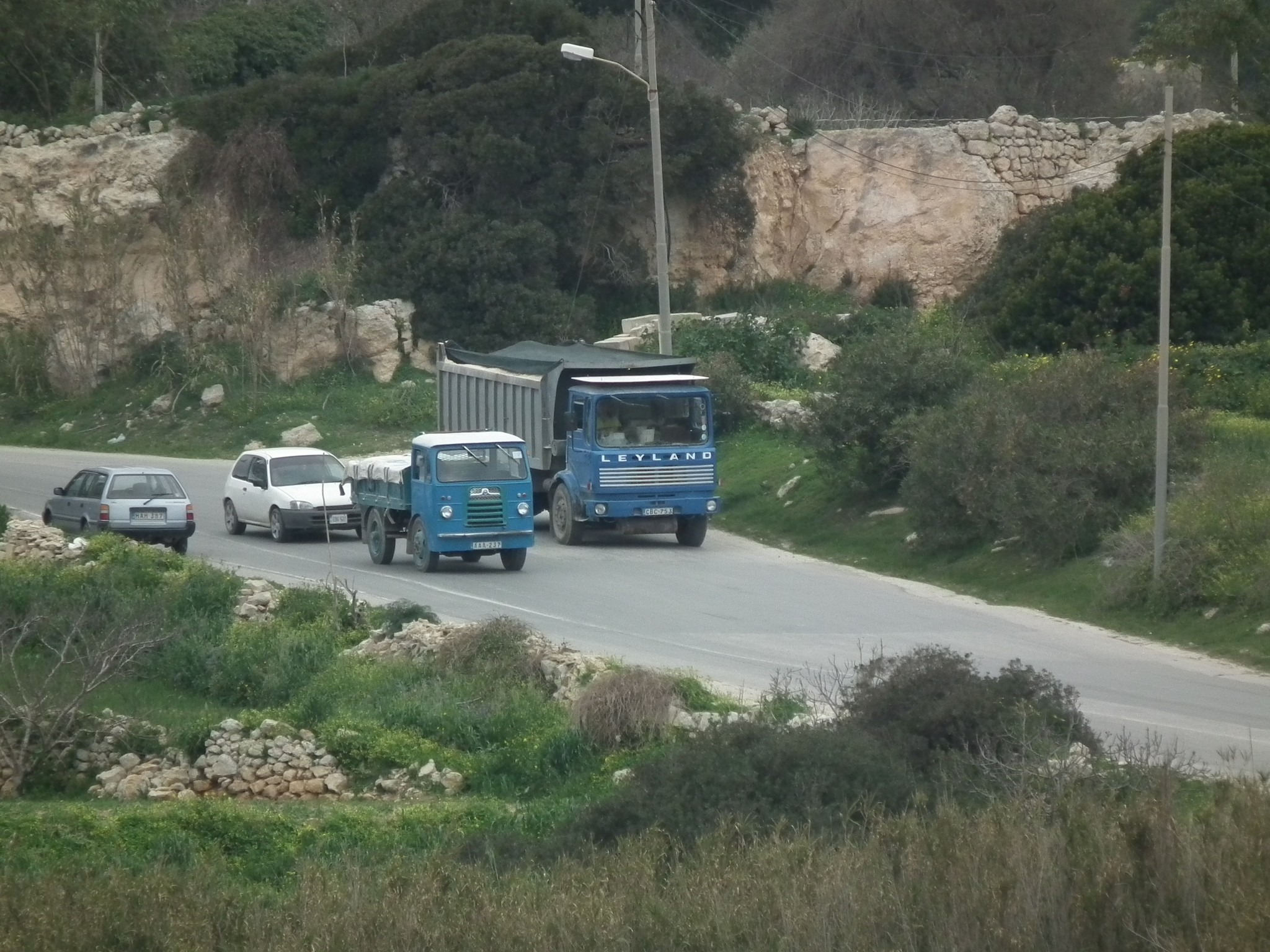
[221,447,363,542]
[41,468,195,557]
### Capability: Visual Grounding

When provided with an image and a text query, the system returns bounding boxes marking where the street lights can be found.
[560,44,673,358]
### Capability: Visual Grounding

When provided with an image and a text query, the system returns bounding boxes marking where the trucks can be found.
[340,431,531,574]
[435,340,719,548]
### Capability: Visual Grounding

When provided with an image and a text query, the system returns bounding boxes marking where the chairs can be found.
[133,483,152,496]
